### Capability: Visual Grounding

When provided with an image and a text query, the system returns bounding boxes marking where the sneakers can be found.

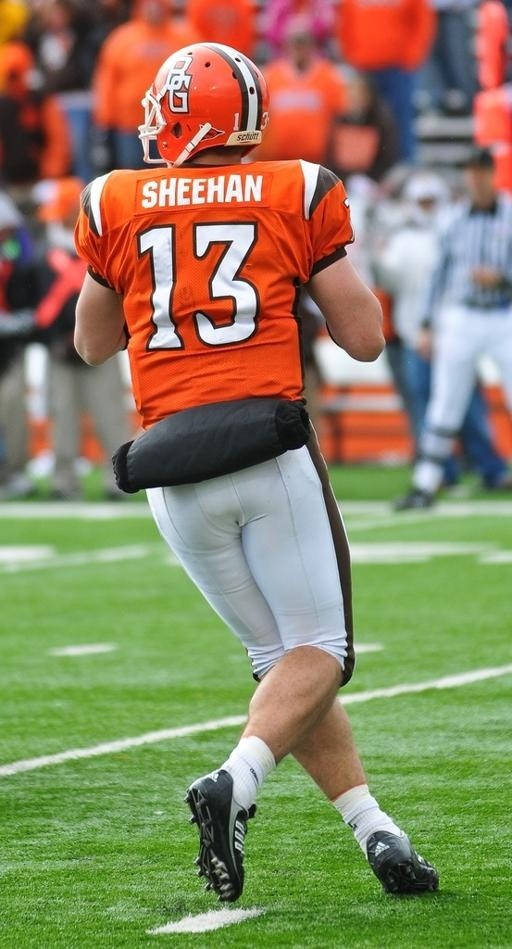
[367,831,439,893]
[185,770,256,902]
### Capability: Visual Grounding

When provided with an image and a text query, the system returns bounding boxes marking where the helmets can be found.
[138,42,270,168]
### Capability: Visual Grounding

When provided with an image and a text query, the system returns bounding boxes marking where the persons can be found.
[65,42,445,907]
[3,4,510,512]
